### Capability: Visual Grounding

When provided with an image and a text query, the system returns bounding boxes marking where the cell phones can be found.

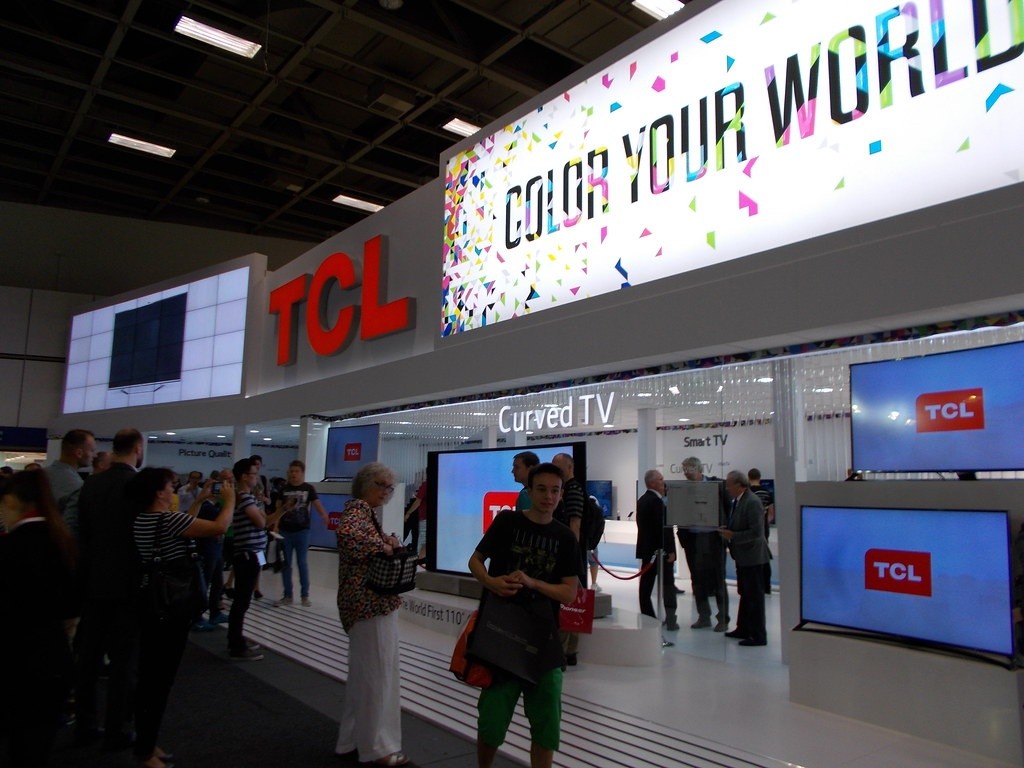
[212,482,224,494]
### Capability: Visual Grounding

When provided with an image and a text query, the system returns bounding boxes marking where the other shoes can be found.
[274,597,294,607]
[692,617,711,629]
[75,726,106,741]
[564,653,578,665]
[244,641,259,650]
[714,621,729,632]
[230,649,264,662]
[223,586,234,599]
[252,590,263,601]
[674,586,685,593]
[192,620,214,632]
[208,613,229,624]
[302,597,312,608]
[725,628,749,639]
[105,732,138,748]
[738,635,766,647]
[139,749,175,767]
[591,581,601,592]
[371,749,410,766]
[667,622,679,631]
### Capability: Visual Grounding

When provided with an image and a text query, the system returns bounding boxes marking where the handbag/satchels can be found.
[371,546,417,595]
[559,577,595,635]
[148,513,204,601]
[449,611,491,690]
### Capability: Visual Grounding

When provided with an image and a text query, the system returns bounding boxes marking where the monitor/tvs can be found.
[308,493,352,550]
[320,424,379,481]
[791,505,1024,671]
[426,440,587,577]
[585,480,614,523]
[850,340,1024,480]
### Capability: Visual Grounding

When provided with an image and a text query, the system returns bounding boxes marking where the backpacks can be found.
[568,483,604,551]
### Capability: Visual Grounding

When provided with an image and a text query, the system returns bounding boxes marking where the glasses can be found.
[373,479,394,494]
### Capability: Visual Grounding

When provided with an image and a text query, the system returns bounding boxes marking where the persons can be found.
[271,459,330,607]
[506,451,599,666]
[332,463,415,768]
[468,461,584,768]
[404,468,427,560]
[0,427,286,768]
[634,456,773,646]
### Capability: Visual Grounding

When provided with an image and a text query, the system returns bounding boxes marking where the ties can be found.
[731,497,738,518]
